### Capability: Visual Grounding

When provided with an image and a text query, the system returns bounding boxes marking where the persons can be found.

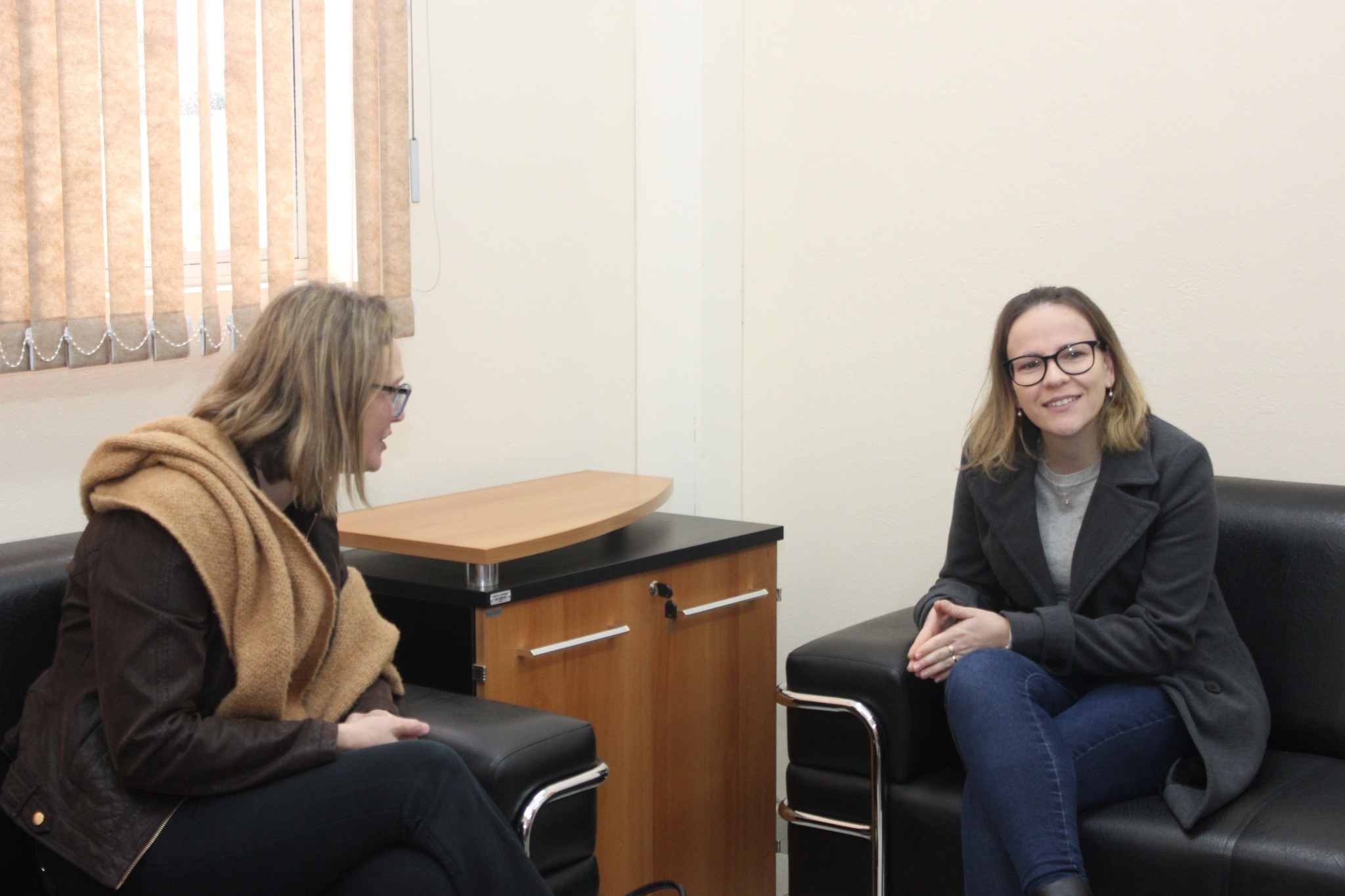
[905,287,1273,896]
[0,283,551,896]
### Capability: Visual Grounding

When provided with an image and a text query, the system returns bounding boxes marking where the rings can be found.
[947,644,954,657]
[953,656,957,663]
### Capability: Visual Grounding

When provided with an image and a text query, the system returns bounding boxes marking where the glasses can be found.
[1008,339,1101,387]
[369,384,411,421]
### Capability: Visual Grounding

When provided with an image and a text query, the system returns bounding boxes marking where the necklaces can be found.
[1038,456,1099,505]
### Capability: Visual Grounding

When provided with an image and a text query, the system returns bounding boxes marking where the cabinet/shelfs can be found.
[337,471,776,896]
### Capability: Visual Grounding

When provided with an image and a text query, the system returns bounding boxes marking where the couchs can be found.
[0,531,608,896]
[787,476,1345,896]
